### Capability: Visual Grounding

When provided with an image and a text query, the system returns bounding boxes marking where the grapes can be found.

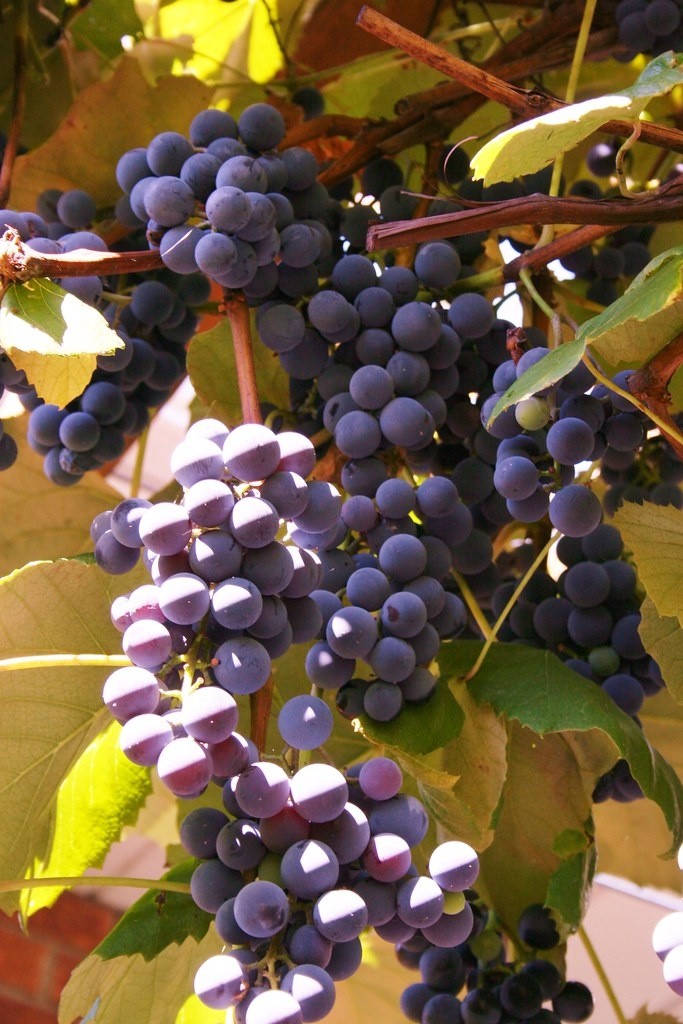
[0,0,683,1024]
